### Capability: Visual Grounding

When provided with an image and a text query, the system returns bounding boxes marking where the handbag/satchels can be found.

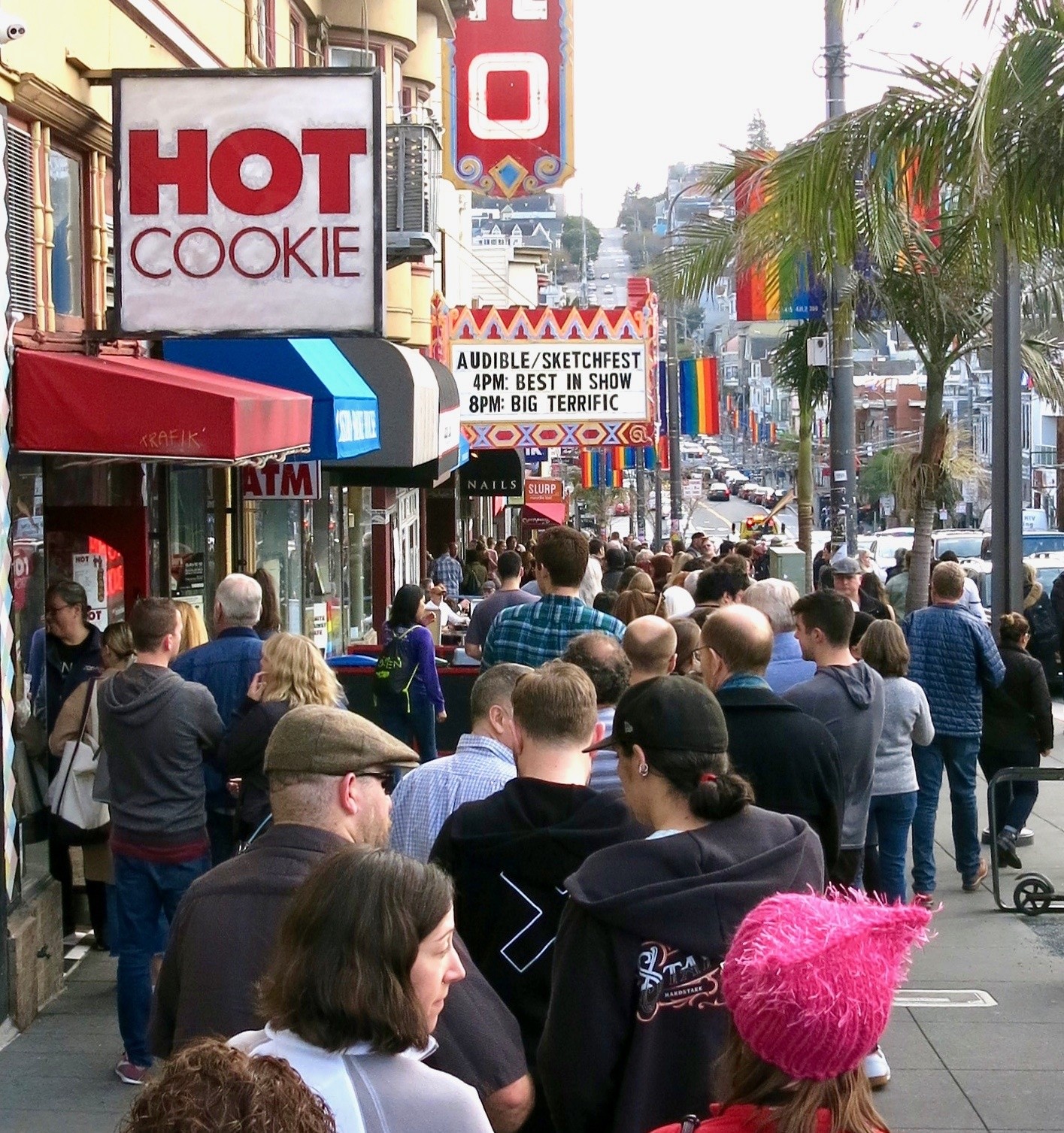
[462,564,481,595]
[1027,603,1057,640]
[51,676,110,830]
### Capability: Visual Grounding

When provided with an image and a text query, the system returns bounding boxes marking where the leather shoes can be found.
[962,859,989,891]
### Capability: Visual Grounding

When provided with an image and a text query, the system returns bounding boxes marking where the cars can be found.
[707,483,730,502]
[613,465,672,520]
[811,508,1064,611]
[586,255,642,306]
[679,434,786,510]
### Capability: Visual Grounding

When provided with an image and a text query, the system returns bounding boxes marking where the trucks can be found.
[731,512,785,541]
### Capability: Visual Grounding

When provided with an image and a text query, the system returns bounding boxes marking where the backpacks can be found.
[372,625,422,712]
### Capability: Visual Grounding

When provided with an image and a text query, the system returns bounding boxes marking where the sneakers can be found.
[861,1045,891,1089]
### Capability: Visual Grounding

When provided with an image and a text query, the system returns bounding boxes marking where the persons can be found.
[1,493,1064,1133]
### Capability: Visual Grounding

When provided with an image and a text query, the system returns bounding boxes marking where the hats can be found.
[830,556,863,575]
[692,532,709,540]
[581,674,729,754]
[722,891,933,1080]
[430,586,447,595]
[754,542,767,557]
[263,704,421,776]
[483,580,496,590]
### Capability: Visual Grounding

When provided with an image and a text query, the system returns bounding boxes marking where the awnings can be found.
[158,337,382,464]
[13,344,313,469]
[320,334,440,470]
[324,356,462,490]
[459,445,526,498]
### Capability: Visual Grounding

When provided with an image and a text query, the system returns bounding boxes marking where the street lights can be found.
[862,390,890,452]
[665,181,726,520]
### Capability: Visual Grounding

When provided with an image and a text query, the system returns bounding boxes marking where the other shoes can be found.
[115,1053,149,1085]
[996,830,1022,869]
[914,892,935,908]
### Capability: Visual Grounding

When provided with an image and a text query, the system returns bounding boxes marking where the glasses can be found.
[48,601,78,615]
[645,590,666,615]
[530,558,537,568]
[691,645,722,662]
[356,767,400,793]
